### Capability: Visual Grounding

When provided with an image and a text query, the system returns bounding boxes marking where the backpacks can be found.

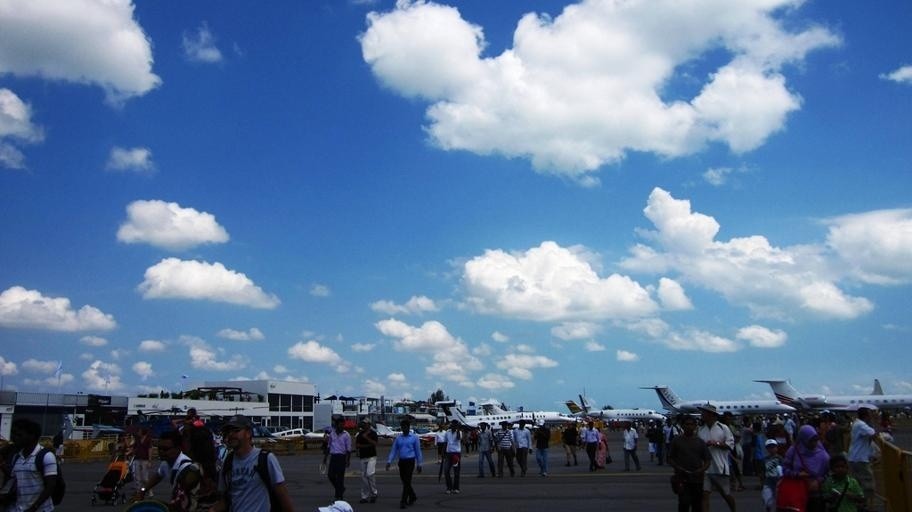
[12,448,66,505]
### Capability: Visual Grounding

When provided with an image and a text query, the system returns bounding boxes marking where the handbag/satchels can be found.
[774,475,807,510]
[605,455,613,465]
[670,476,684,494]
[806,493,825,512]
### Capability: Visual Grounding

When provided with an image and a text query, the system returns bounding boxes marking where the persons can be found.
[666,403,912,512]
[355,417,378,505]
[622,417,685,473]
[559,420,609,472]
[111,407,301,512]
[323,413,351,505]
[0,412,66,511]
[610,418,621,432]
[386,418,423,509]
[434,417,551,494]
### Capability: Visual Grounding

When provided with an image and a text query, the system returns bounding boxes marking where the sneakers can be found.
[444,487,462,496]
[564,460,671,474]
[728,483,763,495]
[476,472,553,479]
[329,494,421,509]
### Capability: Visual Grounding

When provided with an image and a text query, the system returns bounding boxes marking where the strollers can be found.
[89,453,137,508]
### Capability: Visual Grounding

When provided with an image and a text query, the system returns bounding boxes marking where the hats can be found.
[183,414,251,431]
[360,416,371,424]
[763,438,778,447]
[696,404,718,416]
[759,409,836,422]
